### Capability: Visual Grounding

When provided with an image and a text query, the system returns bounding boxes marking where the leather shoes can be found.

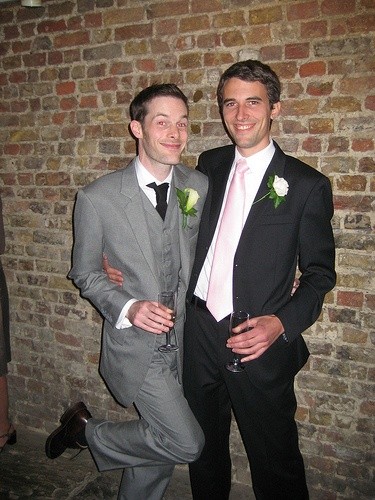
[45,401,92,461]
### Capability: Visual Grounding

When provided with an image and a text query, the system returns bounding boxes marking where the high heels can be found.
[0,423,16,453]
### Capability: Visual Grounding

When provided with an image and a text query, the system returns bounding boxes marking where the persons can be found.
[45,82,211,500]
[0,204,17,452]
[102,60,337,500]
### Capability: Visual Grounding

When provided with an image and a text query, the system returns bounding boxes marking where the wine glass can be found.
[158,292,179,353]
[224,311,249,373]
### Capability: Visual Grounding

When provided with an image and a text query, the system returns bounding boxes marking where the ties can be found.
[206,159,249,323]
[146,182,170,221]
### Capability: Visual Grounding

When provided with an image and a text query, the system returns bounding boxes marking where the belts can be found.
[191,295,231,321]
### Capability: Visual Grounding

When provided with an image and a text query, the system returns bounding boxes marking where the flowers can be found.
[175,187,201,228]
[251,174,289,208]
